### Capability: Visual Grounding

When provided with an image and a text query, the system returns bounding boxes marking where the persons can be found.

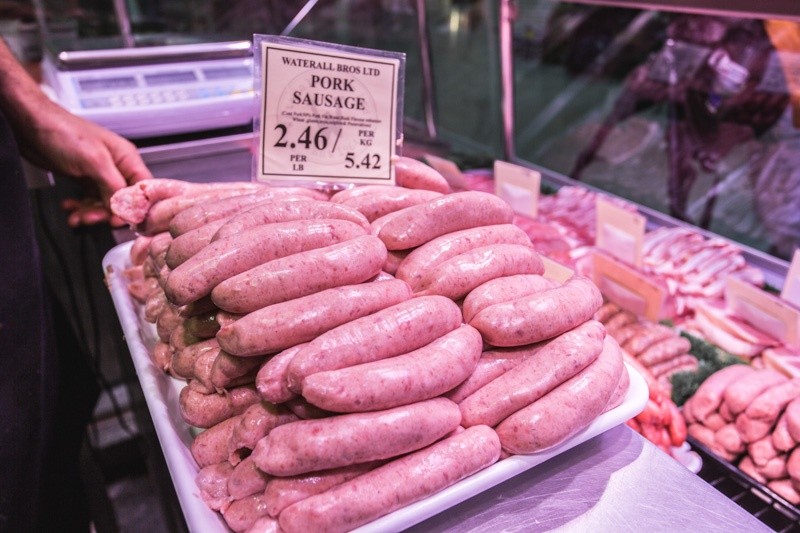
[0,36,158,234]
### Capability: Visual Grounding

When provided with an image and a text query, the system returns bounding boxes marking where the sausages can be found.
[88,128,800,531]
